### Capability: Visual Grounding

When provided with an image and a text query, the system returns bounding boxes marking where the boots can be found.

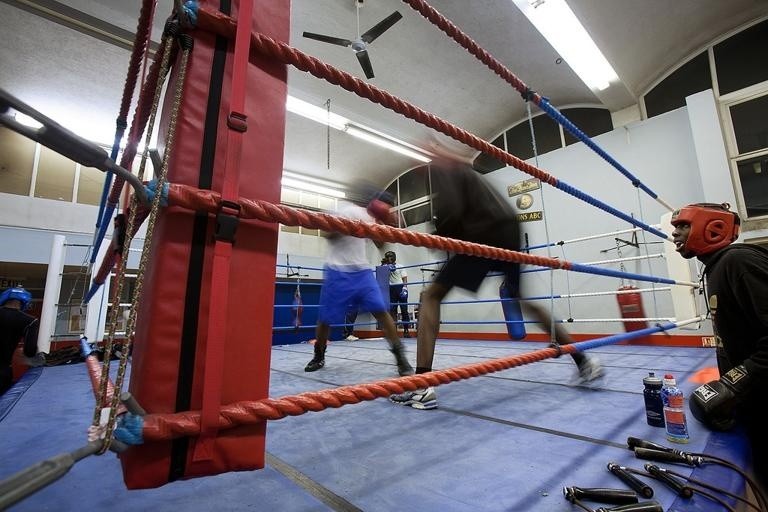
[404,329,411,338]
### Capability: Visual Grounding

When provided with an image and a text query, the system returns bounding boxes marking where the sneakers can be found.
[568,357,604,386]
[389,388,438,409]
[346,335,359,341]
[397,360,414,376]
[305,358,325,372]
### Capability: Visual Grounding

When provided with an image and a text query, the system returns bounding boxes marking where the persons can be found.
[344,302,359,340]
[306,177,415,377]
[0,288,39,397]
[382,251,410,338]
[671,203,768,489]
[390,141,606,409]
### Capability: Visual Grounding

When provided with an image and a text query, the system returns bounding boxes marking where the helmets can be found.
[671,202,740,258]
[0,285,34,312]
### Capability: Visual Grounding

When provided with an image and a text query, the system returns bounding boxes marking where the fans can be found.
[303,0,403,80]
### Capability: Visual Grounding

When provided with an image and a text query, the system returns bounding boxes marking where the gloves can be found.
[367,198,395,226]
[688,364,752,432]
[388,264,396,271]
[400,287,408,299]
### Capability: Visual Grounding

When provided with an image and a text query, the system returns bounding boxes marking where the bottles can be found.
[643,371,690,443]
